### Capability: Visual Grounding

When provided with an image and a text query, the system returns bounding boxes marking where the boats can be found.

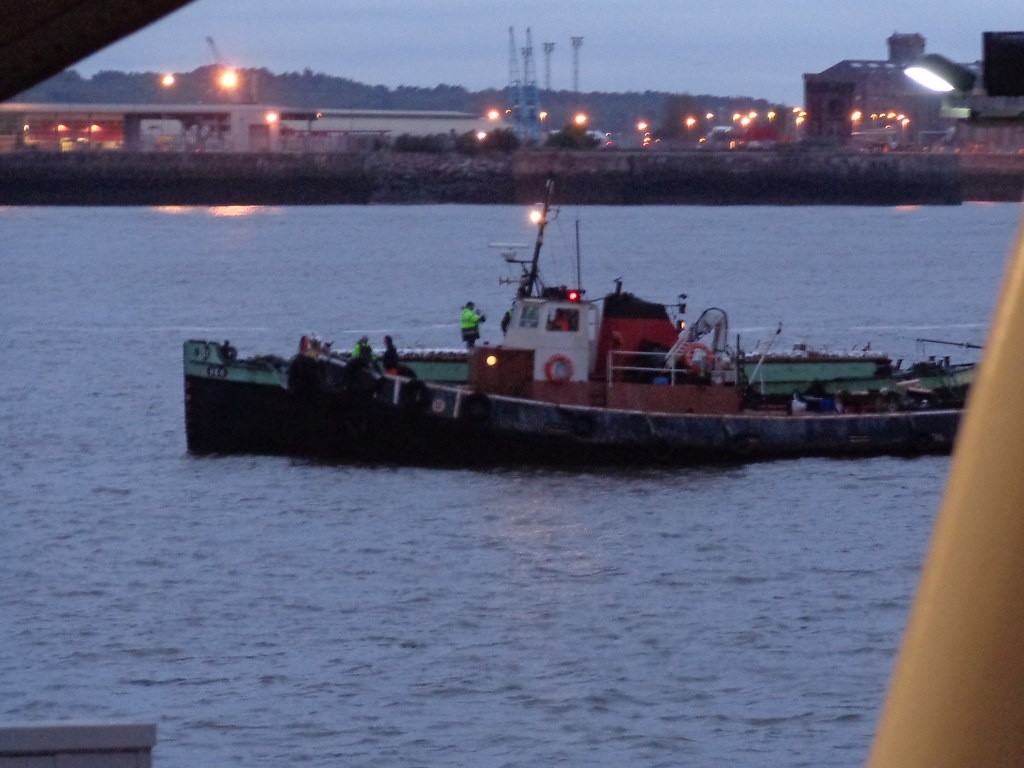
[182,171,985,471]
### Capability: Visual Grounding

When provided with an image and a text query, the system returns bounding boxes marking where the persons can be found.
[350,335,373,364]
[376,336,399,375]
[500,301,516,337]
[460,301,486,350]
[551,308,571,332]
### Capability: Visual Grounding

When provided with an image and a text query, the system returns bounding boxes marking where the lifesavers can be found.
[683,342,714,373]
[544,354,574,385]
